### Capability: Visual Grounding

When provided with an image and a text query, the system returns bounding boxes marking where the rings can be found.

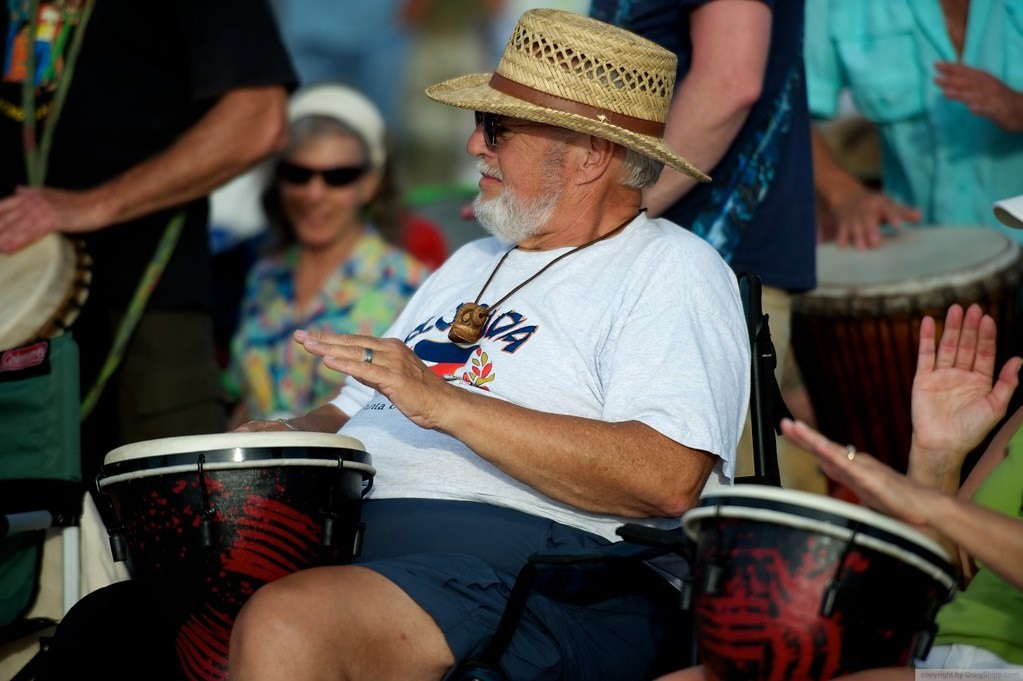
[364,349,373,363]
[847,445,856,461]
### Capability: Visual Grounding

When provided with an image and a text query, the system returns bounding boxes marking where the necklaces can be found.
[448,205,648,343]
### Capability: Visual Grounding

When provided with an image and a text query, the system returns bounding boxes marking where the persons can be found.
[10,6,752,681]
[658,193,1023,681]
[0,0,303,638]
[457,0,821,269]
[802,0,1023,251]
[212,79,451,434]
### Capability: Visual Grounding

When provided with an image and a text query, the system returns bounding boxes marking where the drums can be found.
[790,227,1023,521]
[680,482,963,681]
[0,223,94,352]
[95,432,375,681]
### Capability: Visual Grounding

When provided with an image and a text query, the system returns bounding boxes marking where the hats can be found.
[425,7,713,184]
[286,82,385,170]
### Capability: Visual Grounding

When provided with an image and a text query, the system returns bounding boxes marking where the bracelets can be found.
[276,419,299,431]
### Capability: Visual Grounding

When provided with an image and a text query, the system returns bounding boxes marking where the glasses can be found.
[474,110,564,150]
[275,160,370,187]
